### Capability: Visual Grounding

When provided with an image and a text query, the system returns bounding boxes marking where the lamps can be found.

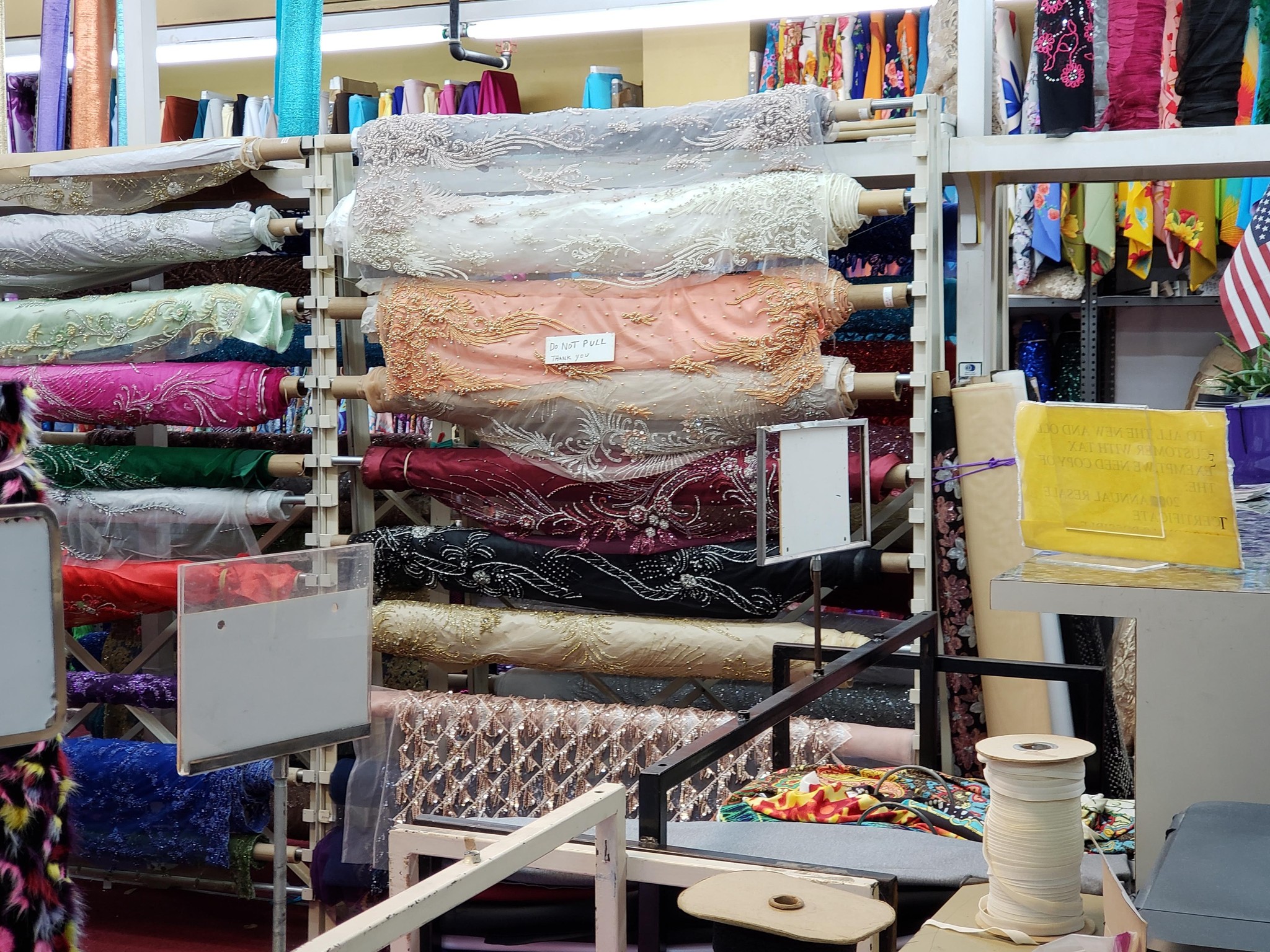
[459,0,939,39]
[4,5,453,80]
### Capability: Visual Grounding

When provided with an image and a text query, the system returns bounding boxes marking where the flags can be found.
[1219,184,1270,352]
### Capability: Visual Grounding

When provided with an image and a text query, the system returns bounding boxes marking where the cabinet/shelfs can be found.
[0,17,1270,402]
